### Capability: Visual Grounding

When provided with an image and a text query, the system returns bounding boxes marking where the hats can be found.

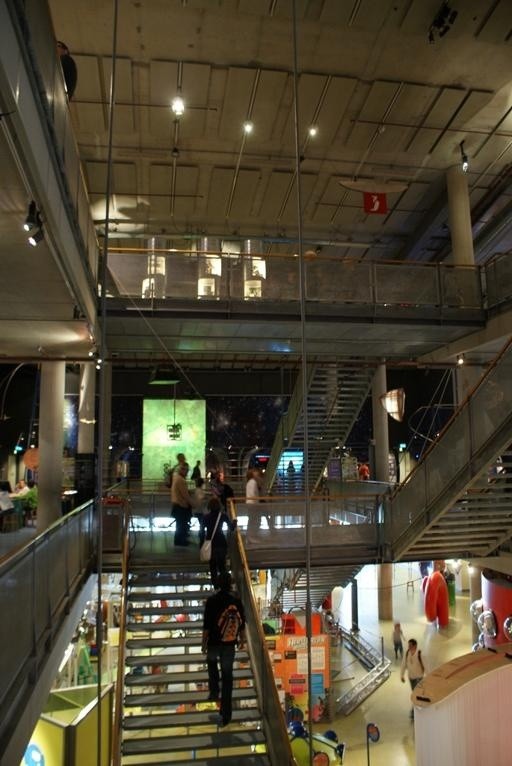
[215,572,233,589]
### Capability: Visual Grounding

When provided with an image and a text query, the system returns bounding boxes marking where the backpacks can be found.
[217,597,242,644]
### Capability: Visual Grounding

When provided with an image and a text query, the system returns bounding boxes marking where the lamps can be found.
[380,387,406,423]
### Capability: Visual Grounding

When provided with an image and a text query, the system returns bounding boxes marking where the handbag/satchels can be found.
[200,537,216,562]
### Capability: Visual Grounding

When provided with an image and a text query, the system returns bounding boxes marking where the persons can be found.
[400,639,428,718]
[392,623,406,659]
[13,479,30,494]
[171,453,261,580]
[201,572,247,726]
[360,463,373,480]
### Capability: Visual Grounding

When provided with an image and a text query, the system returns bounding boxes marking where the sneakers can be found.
[174,523,193,547]
[222,706,232,727]
[208,689,220,702]
[396,655,404,660]
